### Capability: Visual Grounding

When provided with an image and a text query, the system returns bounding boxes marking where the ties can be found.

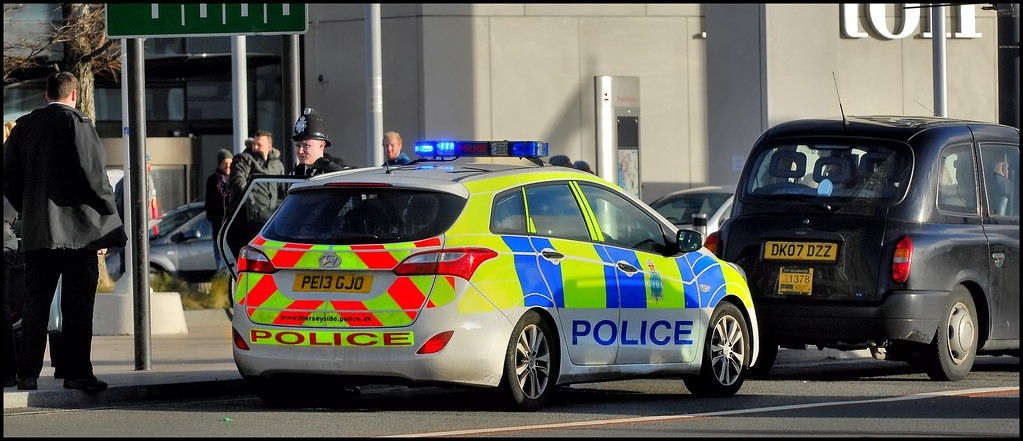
[306,168,312,176]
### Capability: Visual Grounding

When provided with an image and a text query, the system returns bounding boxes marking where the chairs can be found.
[343,197,405,236]
[752,152,896,199]
[957,156,1008,215]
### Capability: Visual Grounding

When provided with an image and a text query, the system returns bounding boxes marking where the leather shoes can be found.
[17,377,37,389]
[63,368,108,391]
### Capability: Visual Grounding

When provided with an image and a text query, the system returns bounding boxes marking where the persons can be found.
[379,131,411,165]
[993,151,1010,176]
[292,108,344,178]
[205,130,286,271]
[115,153,154,223]
[2,71,128,392]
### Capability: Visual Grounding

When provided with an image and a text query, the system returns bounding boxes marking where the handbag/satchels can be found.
[105,252,121,282]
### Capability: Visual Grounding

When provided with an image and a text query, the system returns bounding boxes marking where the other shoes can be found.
[54,366,77,378]
[2,372,18,387]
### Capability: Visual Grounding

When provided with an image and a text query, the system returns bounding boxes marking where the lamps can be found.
[698,31,707,39]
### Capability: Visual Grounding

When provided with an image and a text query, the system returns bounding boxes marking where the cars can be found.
[214,138,760,411]
[640,185,742,255]
[104,201,218,282]
[701,116,1019,383]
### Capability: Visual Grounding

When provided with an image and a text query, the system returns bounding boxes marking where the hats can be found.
[216,149,233,165]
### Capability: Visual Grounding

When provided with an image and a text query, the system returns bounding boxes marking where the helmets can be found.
[291,108,332,147]
